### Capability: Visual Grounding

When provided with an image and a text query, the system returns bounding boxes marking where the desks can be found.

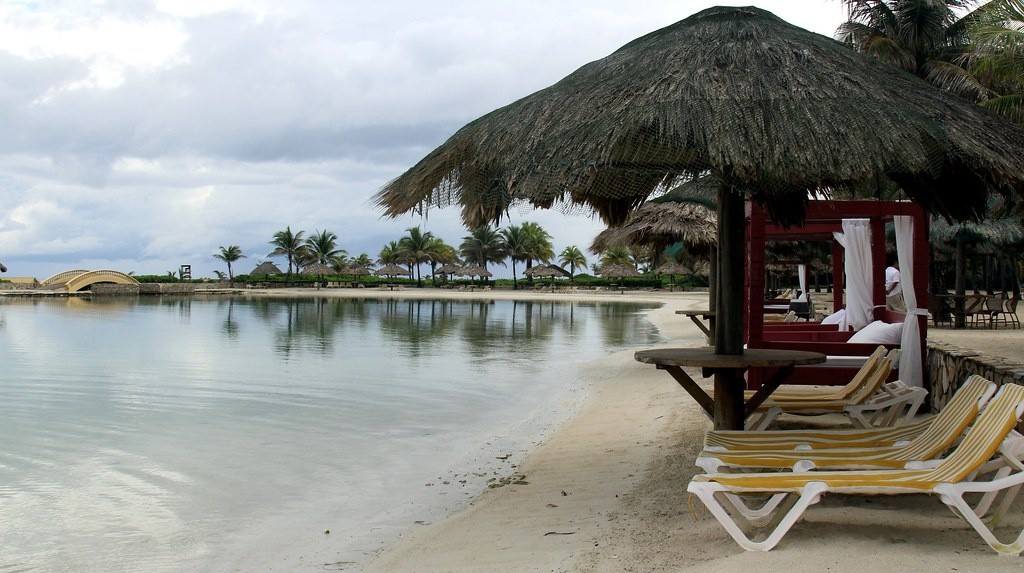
[263,280,677,288]
[634,347,827,431]
[934,293,995,328]
[676,310,716,348]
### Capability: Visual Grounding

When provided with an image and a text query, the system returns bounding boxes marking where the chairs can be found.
[244,281,601,295]
[686,288,1024,558]
[928,290,1022,331]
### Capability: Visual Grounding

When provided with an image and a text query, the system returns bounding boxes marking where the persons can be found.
[885,254,905,311]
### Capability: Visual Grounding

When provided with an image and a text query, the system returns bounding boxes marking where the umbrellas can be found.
[454,264,492,292]
[250,261,283,282]
[434,264,462,282]
[369,7,1024,430]
[535,264,571,292]
[301,263,371,289]
